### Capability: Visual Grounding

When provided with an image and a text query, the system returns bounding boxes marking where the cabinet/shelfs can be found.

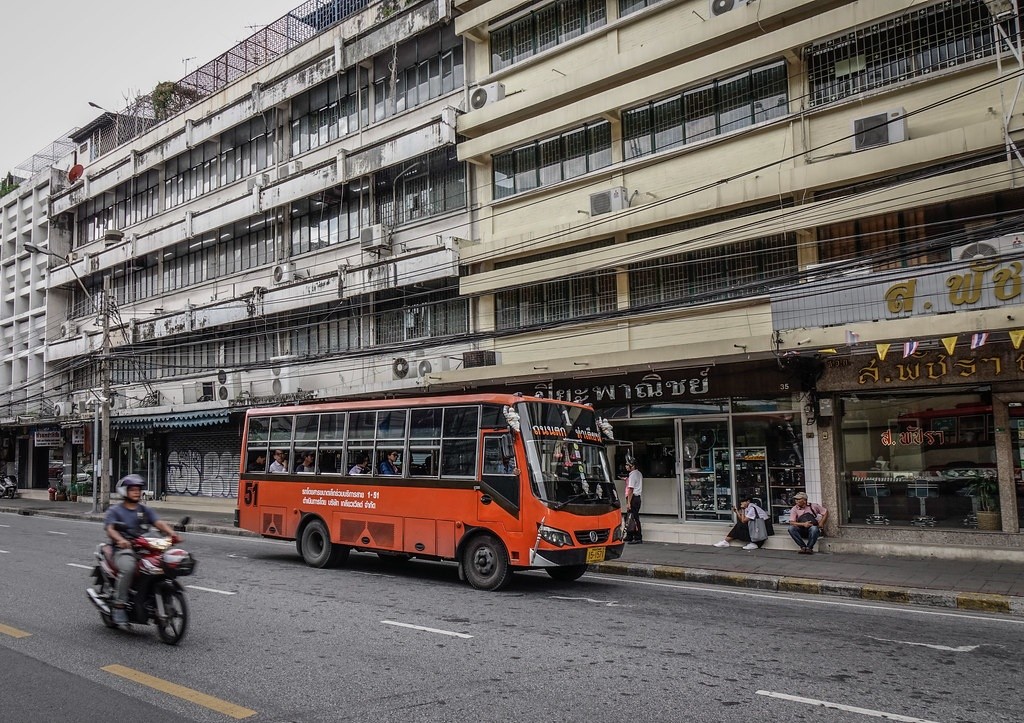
[771,465,807,521]
[713,447,768,514]
[683,470,715,512]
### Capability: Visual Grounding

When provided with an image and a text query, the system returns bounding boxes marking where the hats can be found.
[793,491,808,499]
[627,457,637,469]
[302,451,313,456]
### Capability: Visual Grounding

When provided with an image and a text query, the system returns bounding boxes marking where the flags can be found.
[844,329,1024,360]
[784,349,837,355]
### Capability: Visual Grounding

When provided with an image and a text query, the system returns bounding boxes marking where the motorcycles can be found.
[86,513,197,646]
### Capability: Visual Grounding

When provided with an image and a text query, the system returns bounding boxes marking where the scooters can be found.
[0,474,17,499]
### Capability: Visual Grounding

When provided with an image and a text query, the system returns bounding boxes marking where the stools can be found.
[857,479,892,525]
[905,479,942,528]
[957,487,981,527]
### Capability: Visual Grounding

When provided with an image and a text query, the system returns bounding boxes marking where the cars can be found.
[56,464,91,485]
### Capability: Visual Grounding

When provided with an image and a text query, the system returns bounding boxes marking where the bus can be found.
[233,393,635,592]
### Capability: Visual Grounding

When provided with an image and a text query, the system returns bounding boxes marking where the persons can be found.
[733,495,768,549]
[349,453,371,474]
[103,473,173,623]
[380,451,399,474]
[248,453,266,471]
[295,451,315,471]
[618,458,643,544]
[787,492,828,554]
[497,458,521,475]
[270,450,288,472]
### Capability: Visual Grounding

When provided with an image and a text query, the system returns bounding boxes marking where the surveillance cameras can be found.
[100,397,109,402]
[86,399,93,405]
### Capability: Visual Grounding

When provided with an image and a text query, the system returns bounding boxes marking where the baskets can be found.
[157,556,195,576]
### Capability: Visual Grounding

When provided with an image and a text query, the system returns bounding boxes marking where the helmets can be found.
[165,548,193,569]
[116,473,145,497]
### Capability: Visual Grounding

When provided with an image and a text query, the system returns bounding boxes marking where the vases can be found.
[49,492,55,501]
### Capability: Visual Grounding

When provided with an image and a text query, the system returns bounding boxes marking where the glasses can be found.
[390,453,398,457]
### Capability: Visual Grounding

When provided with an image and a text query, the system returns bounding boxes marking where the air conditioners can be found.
[60,319,77,338]
[360,221,388,249]
[54,402,73,417]
[806,255,873,283]
[247,173,270,191]
[852,107,909,152]
[273,263,296,284]
[469,82,506,111]
[109,390,126,410]
[589,186,629,217]
[278,160,303,179]
[214,368,242,400]
[64,252,78,264]
[417,354,448,376]
[710,0,755,17]
[270,355,299,395]
[391,350,425,379]
[952,233,1023,263]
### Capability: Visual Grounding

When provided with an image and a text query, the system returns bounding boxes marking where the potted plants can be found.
[964,470,1002,529]
[56,484,78,502]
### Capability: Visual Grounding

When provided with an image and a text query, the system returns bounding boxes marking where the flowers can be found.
[48,488,56,493]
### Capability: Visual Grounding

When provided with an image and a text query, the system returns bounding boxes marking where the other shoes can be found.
[799,547,806,554]
[624,536,633,541]
[628,539,642,544]
[743,542,758,549]
[111,608,129,631]
[713,539,729,547]
[806,548,813,553]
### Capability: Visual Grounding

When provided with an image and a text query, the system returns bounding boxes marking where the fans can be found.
[698,428,717,471]
[683,438,700,472]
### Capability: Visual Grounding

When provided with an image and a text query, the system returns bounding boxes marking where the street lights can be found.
[23,242,111,510]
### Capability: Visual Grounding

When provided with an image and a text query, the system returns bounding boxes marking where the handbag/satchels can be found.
[622,509,639,533]
[798,512,819,528]
[748,506,768,542]
